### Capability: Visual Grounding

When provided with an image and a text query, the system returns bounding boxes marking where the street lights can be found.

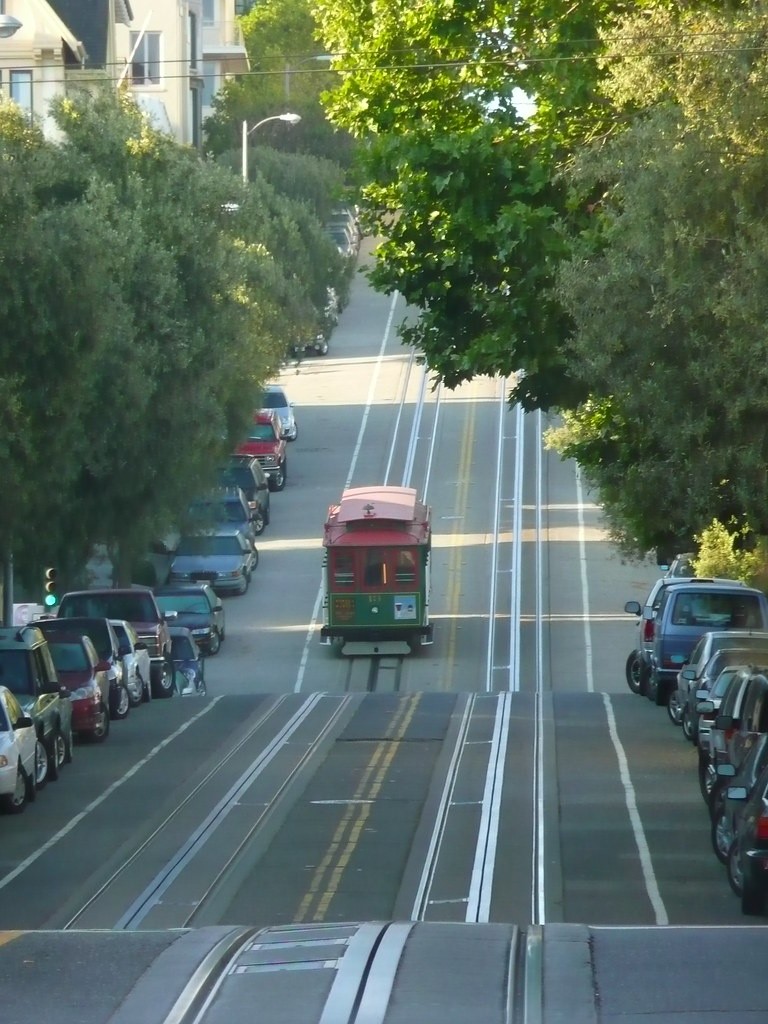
[286,56,336,107]
[242,113,303,189]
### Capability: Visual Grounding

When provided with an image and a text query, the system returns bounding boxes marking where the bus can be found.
[319,486,435,657]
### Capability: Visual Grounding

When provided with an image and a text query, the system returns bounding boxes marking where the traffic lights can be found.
[41,565,58,609]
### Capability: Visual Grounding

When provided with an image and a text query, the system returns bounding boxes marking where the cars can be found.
[1,195,362,786]
[623,553,767,916]
[0,685,40,816]
